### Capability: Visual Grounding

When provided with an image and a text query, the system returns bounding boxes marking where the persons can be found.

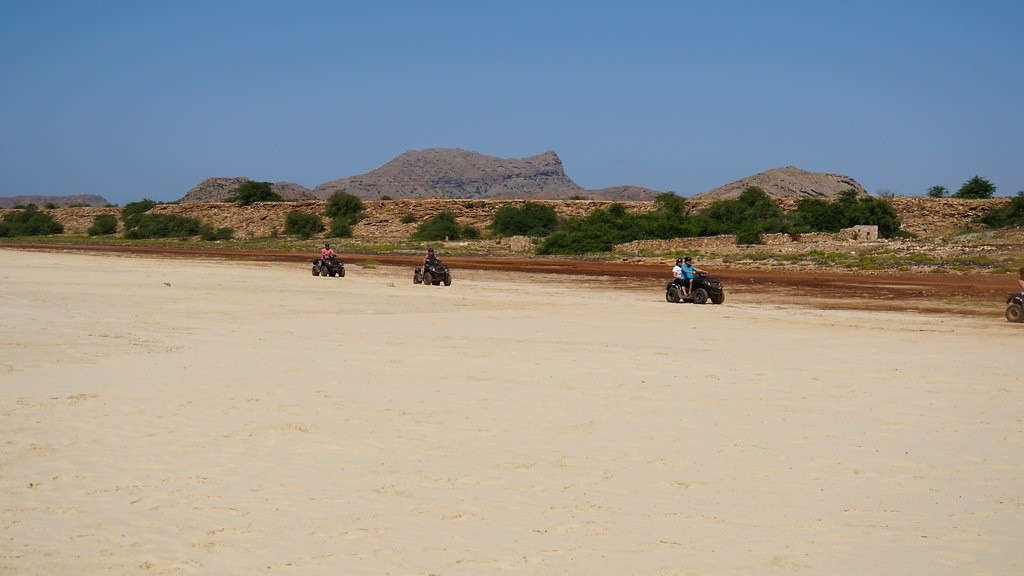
[422,248,439,277]
[1018,268,1024,290]
[672,257,707,296]
[321,243,337,265]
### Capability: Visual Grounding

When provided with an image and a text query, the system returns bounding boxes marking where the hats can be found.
[677,258,683,262]
[428,248,433,252]
[685,257,691,261]
[325,243,330,248]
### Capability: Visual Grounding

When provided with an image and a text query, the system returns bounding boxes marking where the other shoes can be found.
[685,293,688,297]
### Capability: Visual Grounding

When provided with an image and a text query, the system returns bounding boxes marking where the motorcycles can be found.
[666,271,725,304]
[413,258,451,286]
[312,253,345,277]
[1006,280,1024,322]
